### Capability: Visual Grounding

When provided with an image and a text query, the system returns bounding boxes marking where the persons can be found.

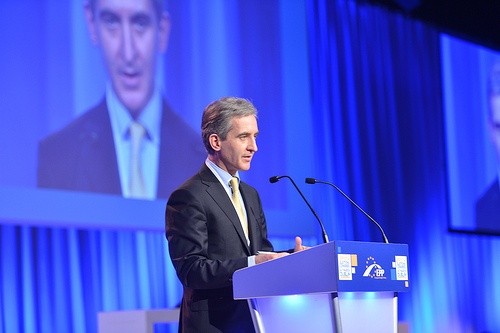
[163,96,314,333]
[475,68,500,230]
[38,0,211,202]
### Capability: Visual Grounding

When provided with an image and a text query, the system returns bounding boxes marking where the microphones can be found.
[269,176,328,245]
[305,178,388,243]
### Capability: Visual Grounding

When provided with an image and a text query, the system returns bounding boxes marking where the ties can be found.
[230,177,250,246]
[129,123,146,198]
